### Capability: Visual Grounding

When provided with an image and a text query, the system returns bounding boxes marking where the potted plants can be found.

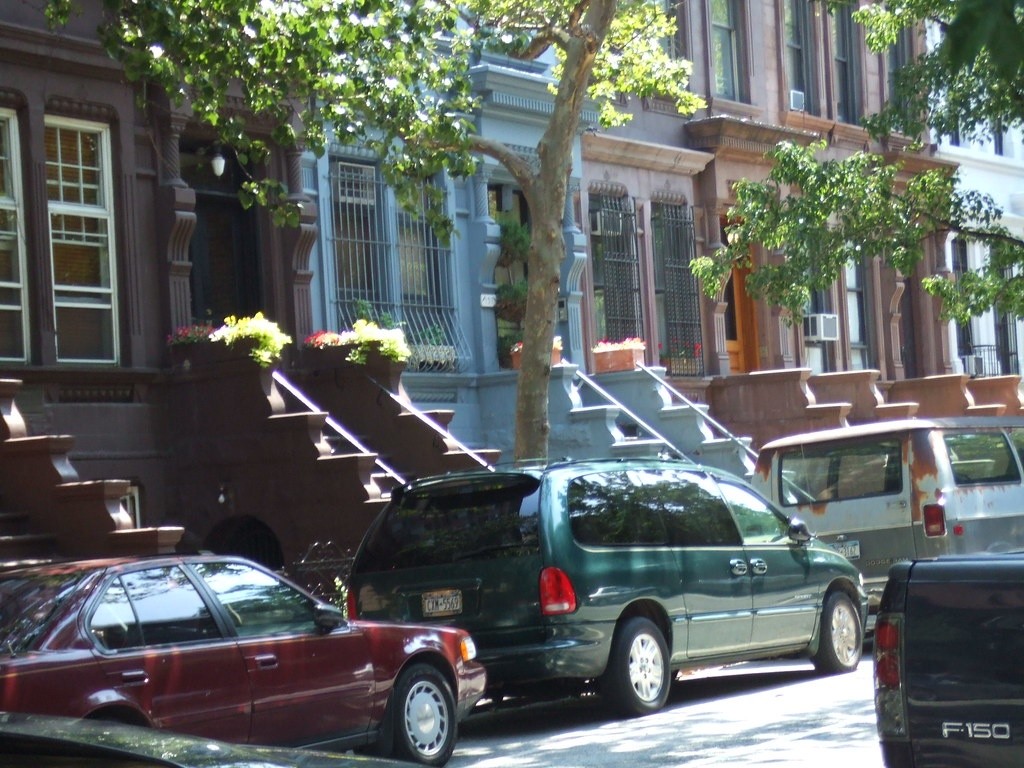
[409,321,458,365]
[209,311,292,368]
[340,318,412,370]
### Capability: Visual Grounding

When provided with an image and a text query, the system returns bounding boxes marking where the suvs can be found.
[337,455,870,713]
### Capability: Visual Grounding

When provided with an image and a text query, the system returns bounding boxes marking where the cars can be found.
[0,552,490,768]
[874,550,1024,766]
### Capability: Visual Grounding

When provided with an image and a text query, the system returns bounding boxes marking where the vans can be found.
[751,417,1024,612]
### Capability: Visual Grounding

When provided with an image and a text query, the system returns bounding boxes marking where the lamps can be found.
[210,140,228,181]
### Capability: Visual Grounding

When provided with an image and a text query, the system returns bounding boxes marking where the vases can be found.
[170,343,216,367]
[511,349,562,370]
[594,349,645,375]
[659,357,702,376]
[303,348,345,369]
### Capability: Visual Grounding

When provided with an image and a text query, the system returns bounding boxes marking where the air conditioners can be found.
[788,88,807,112]
[803,312,840,343]
[961,354,986,378]
[589,209,623,238]
[329,161,379,207]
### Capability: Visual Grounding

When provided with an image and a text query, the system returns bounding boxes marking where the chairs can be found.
[121,588,204,648]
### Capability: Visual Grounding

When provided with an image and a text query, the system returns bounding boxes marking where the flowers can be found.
[656,337,702,360]
[509,336,563,355]
[164,323,219,348]
[302,328,345,351]
[592,337,647,353]
